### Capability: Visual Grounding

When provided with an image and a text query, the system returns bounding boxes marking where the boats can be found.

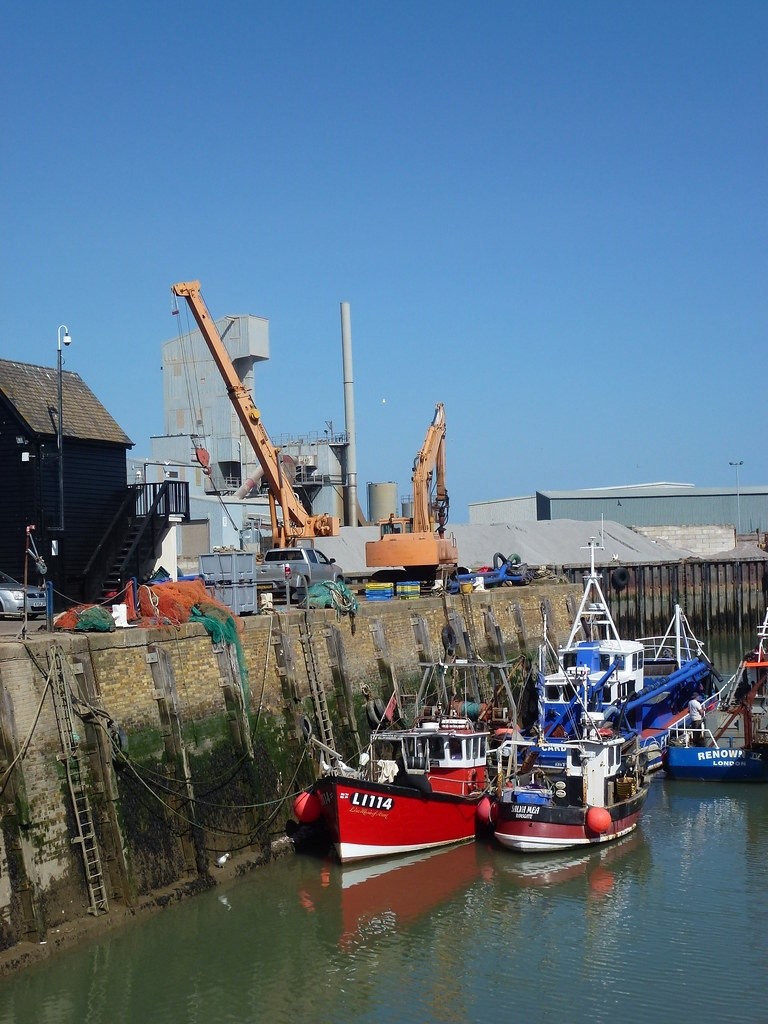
[291,511,768,869]
[309,838,478,958]
[488,827,643,894]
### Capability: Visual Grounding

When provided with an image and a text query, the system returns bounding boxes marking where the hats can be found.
[691,692,698,699]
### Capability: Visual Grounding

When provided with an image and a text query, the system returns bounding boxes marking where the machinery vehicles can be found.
[364,402,458,598]
[170,279,342,556]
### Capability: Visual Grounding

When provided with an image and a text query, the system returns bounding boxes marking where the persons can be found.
[688,692,707,747]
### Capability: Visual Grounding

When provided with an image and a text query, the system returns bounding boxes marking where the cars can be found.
[0,571,47,620]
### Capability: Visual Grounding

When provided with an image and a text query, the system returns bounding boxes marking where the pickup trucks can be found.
[255,546,346,604]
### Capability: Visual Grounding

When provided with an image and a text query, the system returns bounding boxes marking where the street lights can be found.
[21,323,72,587]
[729,461,744,533]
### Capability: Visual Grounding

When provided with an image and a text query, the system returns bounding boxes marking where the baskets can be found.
[616,776,637,797]
